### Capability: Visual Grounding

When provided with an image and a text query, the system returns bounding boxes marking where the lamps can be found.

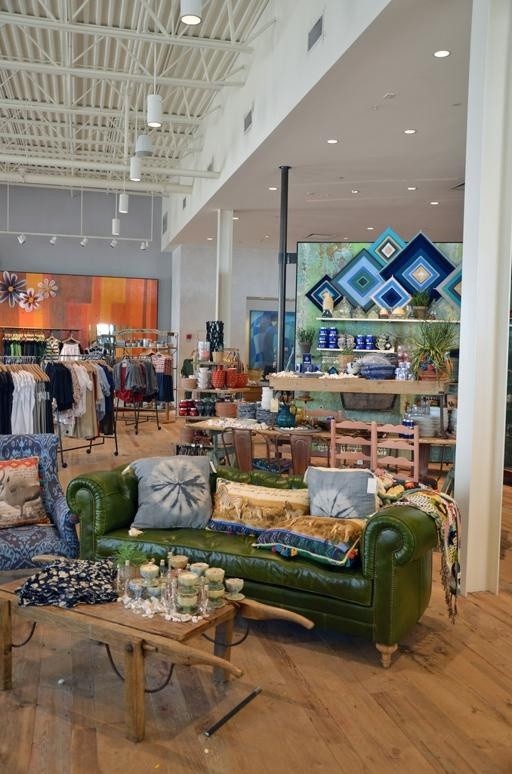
[110,40,164,238]
[177,0,204,27]
[16,231,150,252]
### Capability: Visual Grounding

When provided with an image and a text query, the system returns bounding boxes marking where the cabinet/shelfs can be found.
[312,309,461,372]
[175,359,252,428]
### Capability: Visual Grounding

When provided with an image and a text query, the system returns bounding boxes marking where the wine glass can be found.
[195,397,216,416]
[117,554,226,617]
[226,577,246,601]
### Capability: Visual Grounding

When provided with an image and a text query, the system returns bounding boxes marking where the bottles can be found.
[180,399,198,416]
[339,295,416,319]
[277,401,295,427]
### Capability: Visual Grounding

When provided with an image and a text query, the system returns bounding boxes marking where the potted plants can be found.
[336,343,355,369]
[402,311,458,382]
[412,285,434,321]
[294,325,315,354]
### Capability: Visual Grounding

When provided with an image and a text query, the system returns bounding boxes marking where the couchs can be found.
[65,452,441,671]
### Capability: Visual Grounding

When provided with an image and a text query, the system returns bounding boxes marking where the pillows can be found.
[0,456,48,529]
[252,513,369,572]
[299,459,380,519]
[203,472,310,536]
[125,454,219,531]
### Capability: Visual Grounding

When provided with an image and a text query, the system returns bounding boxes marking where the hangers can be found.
[0,356,115,380]
[2,324,82,346]
[114,348,175,362]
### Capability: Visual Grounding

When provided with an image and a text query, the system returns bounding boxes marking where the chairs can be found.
[219,406,422,483]
[0,431,83,578]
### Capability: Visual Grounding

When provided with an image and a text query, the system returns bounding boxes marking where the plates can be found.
[399,404,457,437]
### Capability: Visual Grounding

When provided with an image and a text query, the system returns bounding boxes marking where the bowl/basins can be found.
[359,362,395,379]
[237,401,274,424]
[216,402,235,417]
[182,317,264,390]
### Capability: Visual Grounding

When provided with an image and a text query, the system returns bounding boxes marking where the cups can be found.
[394,361,415,380]
[374,334,392,349]
[338,336,355,348]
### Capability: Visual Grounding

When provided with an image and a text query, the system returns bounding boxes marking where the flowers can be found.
[0,270,58,314]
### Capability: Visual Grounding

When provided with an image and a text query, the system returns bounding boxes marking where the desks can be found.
[1,551,316,744]
[183,415,459,481]
[268,373,453,440]
